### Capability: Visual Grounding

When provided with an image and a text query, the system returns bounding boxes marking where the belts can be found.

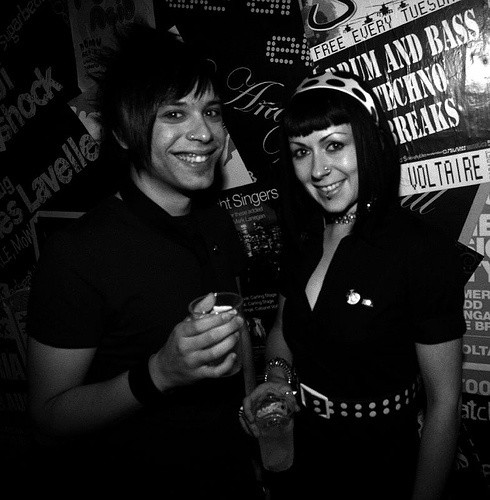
[291,368,421,420]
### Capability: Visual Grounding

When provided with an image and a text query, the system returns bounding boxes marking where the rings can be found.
[238,406,243,416]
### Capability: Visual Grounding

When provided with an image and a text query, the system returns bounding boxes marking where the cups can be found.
[250,396,294,473]
[188,293,244,378]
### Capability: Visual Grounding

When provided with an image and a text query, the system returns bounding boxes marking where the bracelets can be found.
[264,357,293,385]
[128,354,162,403]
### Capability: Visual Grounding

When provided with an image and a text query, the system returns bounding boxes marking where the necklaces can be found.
[334,213,356,224]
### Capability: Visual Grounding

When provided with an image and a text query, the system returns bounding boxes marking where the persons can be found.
[28,51,260,500]
[236,71,467,500]
